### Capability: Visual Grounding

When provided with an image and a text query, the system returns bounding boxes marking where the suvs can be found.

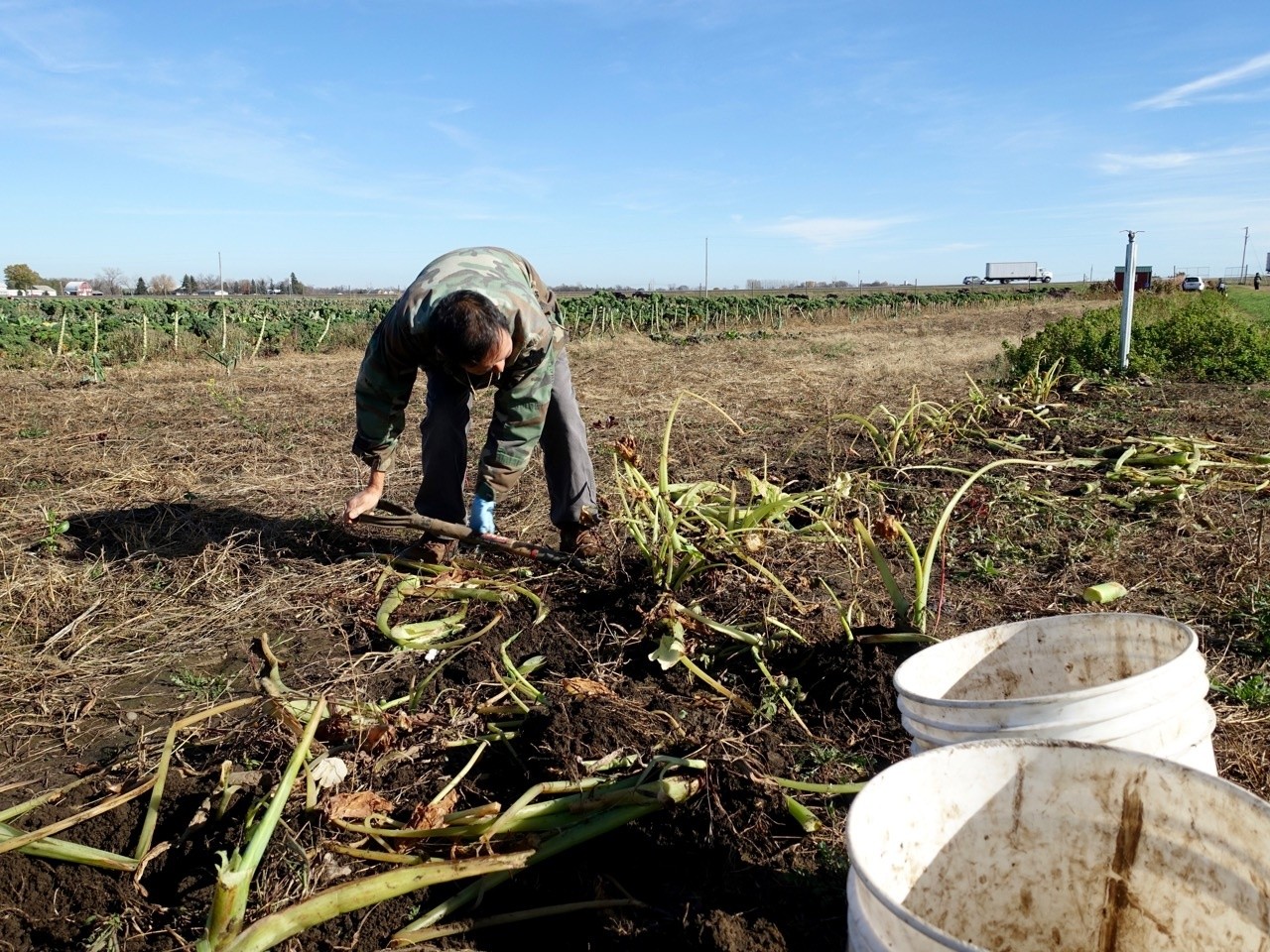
[963,276,987,285]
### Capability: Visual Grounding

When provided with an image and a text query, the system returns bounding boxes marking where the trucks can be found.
[1183,276,1205,291]
[984,261,1053,283]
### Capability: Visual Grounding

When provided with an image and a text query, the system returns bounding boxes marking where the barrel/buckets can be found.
[841,737,1269,952]
[893,611,1222,781]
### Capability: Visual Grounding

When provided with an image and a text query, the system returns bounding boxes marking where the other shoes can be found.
[560,528,599,557]
[411,531,450,567]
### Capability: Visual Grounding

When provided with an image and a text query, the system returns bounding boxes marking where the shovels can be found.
[352,498,607,597]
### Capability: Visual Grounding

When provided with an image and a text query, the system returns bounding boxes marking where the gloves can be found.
[462,499,497,547]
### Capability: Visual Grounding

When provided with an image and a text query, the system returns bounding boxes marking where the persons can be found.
[342,246,600,564]
[1216,278,1225,294]
[1254,273,1262,290]
